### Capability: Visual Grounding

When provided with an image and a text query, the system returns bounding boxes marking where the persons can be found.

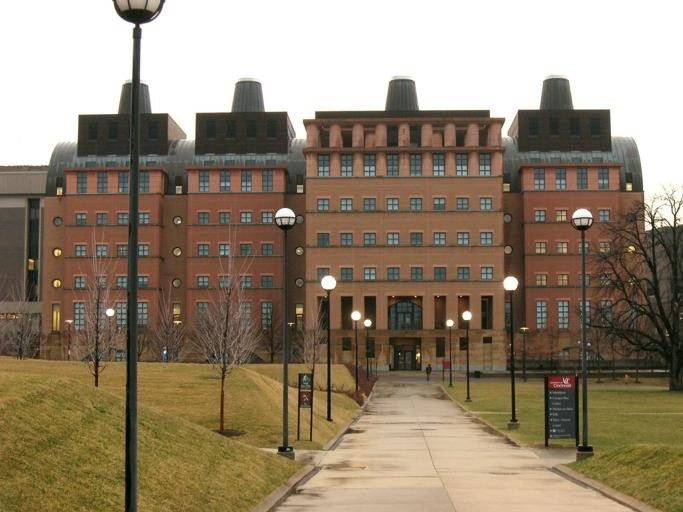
[161,346,177,362]
[426,364,432,381]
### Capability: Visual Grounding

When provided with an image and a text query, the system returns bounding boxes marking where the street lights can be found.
[273,207,297,452]
[502,276,518,422]
[105,0,167,510]
[570,209,595,450]
[320,275,336,420]
[351,311,372,390]
[446,310,473,399]
